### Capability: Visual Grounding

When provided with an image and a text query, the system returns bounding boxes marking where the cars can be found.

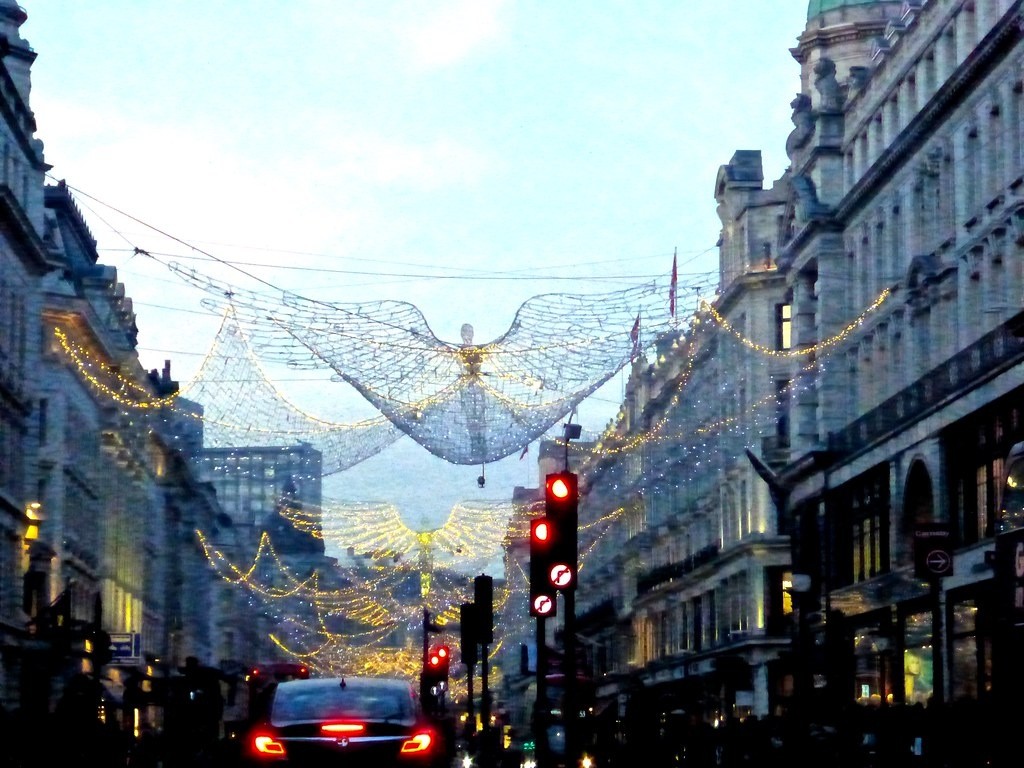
[241,670,449,768]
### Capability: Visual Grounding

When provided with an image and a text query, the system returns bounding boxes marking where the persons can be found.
[163,656,250,768]
[467,717,525,768]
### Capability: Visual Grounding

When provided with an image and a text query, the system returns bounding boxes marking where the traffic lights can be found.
[426,654,442,696]
[544,471,581,593]
[435,646,450,694]
[530,517,557,618]
